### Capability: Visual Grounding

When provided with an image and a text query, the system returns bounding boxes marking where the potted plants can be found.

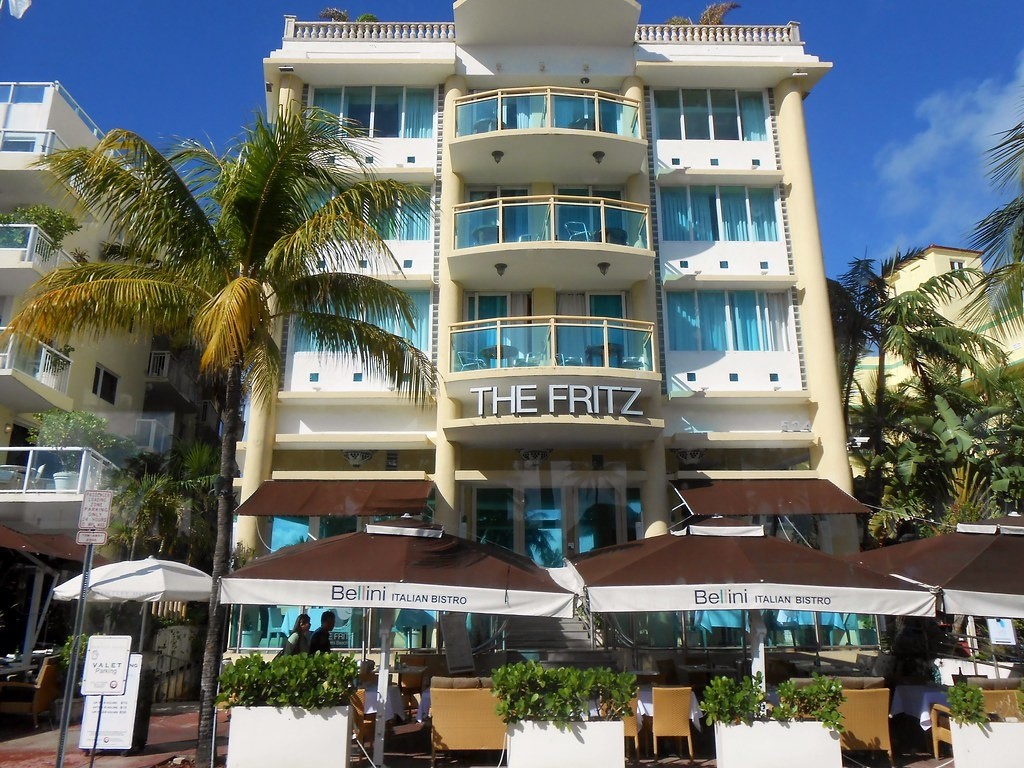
[0,200,130,493]
[211,649,361,767]
[237,622,263,649]
[697,670,847,768]
[55,631,102,723]
[491,660,640,768]
[940,679,1024,767]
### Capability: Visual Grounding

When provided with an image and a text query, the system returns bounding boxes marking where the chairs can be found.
[0,654,62,731]
[453,341,584,373]
[564,220,590,242]
[267,607,285,648]
[338,670,694,763]
[19,464,45,490]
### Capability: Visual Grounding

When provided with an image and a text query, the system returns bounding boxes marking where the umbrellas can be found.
[51,555,212,651]
[844,511,1024,676]
[219,512,582,768]
[562,514,944,718]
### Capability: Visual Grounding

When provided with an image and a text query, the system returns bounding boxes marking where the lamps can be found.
[488,149,610,276]
[339,447,706,469]
[4,422,13,433]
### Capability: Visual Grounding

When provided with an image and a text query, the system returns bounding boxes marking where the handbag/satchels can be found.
[873,648,903,689]
[271,632,300,662]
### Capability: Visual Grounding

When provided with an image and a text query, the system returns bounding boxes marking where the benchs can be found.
[930,678,1022,761]
[430,675,510,766]
[780,675,894,768]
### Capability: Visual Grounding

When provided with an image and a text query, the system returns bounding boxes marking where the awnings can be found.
[668,478,873,515]
[234,479,433,518]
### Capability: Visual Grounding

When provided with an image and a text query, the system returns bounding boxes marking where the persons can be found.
[283,611,336,656]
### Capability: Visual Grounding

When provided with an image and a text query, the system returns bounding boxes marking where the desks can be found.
[890,684,952,754]
[546,682,704,757]
[480,345,516,368]
[356,681,406,746]
[585,341,624,367]
[469,113,628,244]
[0,464,35,490]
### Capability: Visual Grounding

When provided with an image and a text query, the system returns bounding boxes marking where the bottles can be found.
[815,651,821,666]
[394,651,400,669]
[958,667,964,683]
